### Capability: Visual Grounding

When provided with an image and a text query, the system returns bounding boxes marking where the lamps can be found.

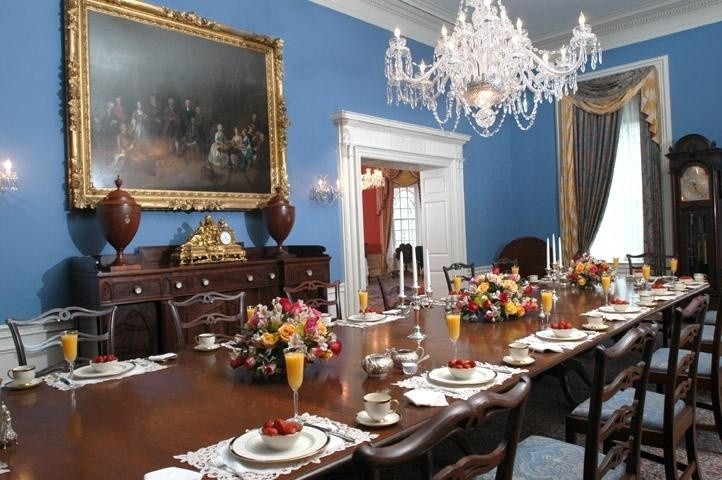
[311,175,341,205]
[382,0,603,137]
[1,156,22,193]
[360,167,386,190]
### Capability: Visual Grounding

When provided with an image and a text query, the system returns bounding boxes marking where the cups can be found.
[321,313,331,324]
[60,330,78,380]
[639,294,653,303]
[693,273,707,281]
[586,313,606,326]
[7,365,36,383]
[527,275,539,282]
[363,393,400,422]
[403,363,417,376]
[508,343,535,361]
[195,333,215,347]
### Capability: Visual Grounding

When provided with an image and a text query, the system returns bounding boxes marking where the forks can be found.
[208,450,242,479]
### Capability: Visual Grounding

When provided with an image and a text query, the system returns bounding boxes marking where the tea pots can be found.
[360,348,393,379]
[388,347,424,368]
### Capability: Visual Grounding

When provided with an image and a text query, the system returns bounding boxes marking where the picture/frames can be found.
[61,0,287,213]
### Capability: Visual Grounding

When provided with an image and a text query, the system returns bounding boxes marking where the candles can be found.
[412,244,418,287]
[399,250,405,298]
[558,236,562,268]
[546,238,550,271]
[426,249,431,294]
[552,234,557,264]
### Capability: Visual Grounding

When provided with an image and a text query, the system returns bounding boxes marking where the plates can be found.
[229,424,330,463]
[582,323,609,330]
[503,356,535,365]
[636,302,658,307]
[529,280,541,284]
[348,313,386,321]
[73,362,136,376]
[354,410,400,428]
[427,367,497,387]
[323,322,336,328]
[598,305,641,313]
[5,378,42,389]
[536,329,588,340]
[195,344,220,351]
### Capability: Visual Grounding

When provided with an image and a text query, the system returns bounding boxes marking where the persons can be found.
[93,96,269,172]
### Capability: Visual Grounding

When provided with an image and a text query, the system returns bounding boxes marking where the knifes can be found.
[304,423,356,444]
[50,372,70,385]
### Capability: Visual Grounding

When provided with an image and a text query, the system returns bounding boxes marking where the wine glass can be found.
[642,264,651,289]
[285,349,305,425]
[358,291,369,329]
[446,310,461,365]
[454,276,461,294]
[601,274,611,306]
[247,305,255,319]
[540,290,553,329]
[613,257,619,275]
[670,258,678,284]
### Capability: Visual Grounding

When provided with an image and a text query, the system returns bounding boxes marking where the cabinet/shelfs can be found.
[69,258,329,361]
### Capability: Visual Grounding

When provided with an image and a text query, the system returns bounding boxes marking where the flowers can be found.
[453,266,540,324]
[225,295,342,379]
[563,251,616,291]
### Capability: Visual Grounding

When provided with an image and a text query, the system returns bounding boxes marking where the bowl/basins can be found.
[552,329,574,338]
[612,304,629,311]
[89,358,118,372]
[680,278,692,284]
[448,367,476,380]
[258,422,302,451]
[651,287,668,295]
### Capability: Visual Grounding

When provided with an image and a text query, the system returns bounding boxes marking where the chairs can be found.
[626,253,680,279]
[168,292,245,352]
[492,258,518,275]
[6,305,118,378]
[376,270,413,311]
[282,279,342,322]
[442,263,475,294]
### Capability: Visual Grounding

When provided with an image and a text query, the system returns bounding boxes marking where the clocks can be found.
[664,132,721,310]
